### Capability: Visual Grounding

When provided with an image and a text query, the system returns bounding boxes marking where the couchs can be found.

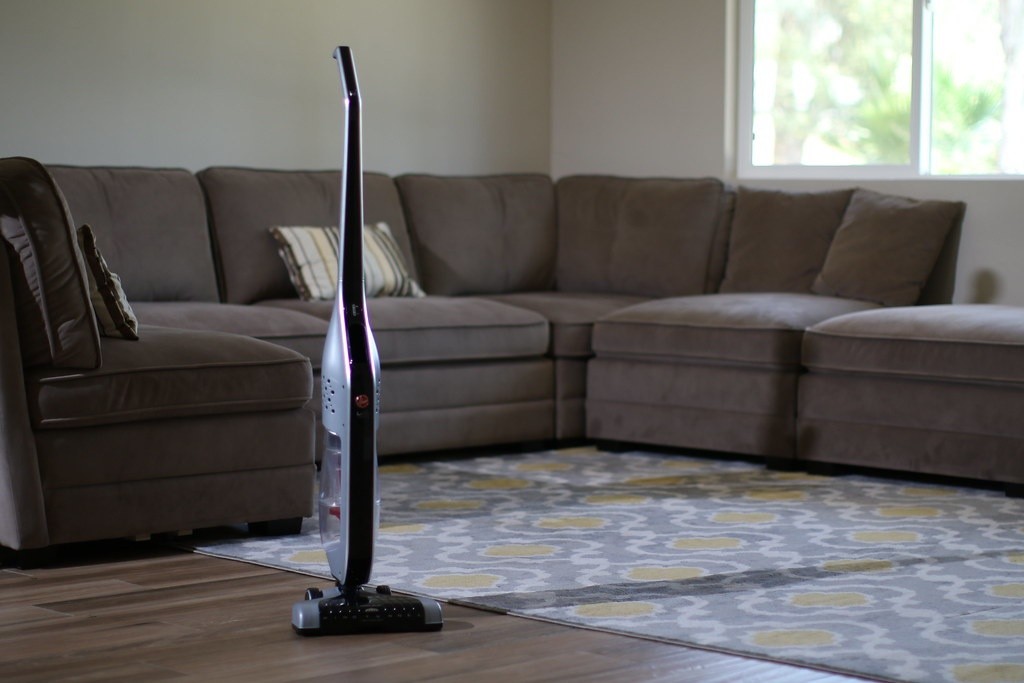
[0,152,1023,565]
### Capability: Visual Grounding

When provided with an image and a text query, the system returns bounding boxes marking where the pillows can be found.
[270,221,428,300]
[719,186,853,292]
[76,223,141,342]
[811,188,959,305]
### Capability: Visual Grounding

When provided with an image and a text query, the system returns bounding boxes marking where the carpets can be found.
[159,445,1024,682]
